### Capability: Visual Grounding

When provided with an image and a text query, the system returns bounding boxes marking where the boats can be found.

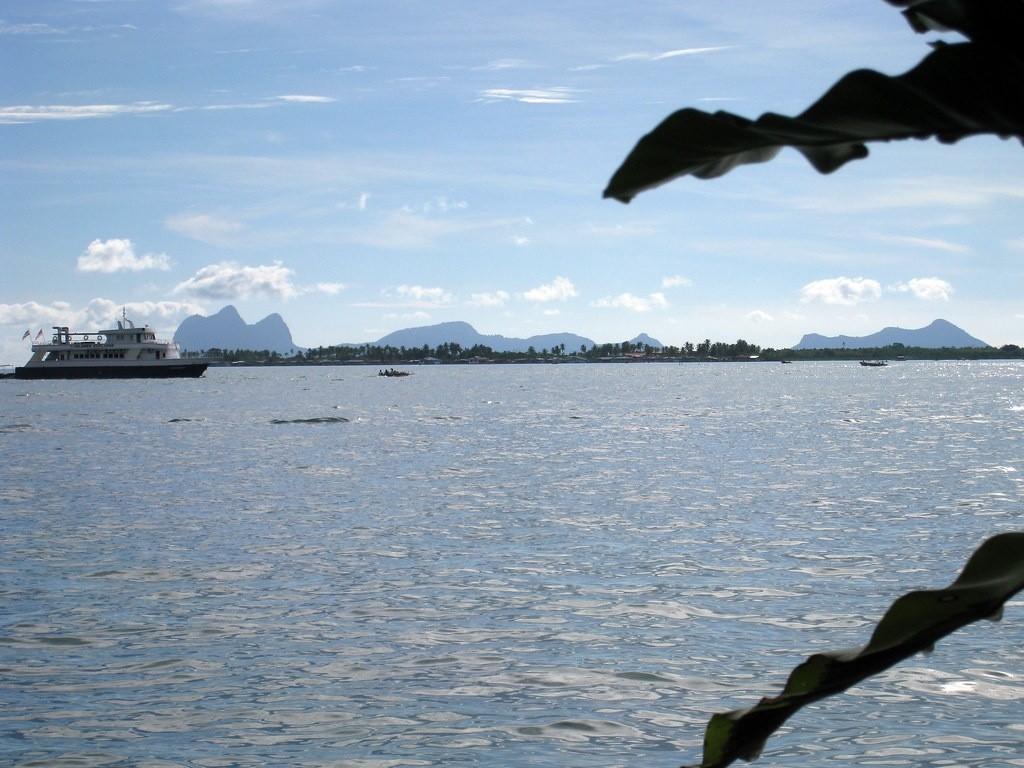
[860,358,889,367]
[15,306,212,380]
